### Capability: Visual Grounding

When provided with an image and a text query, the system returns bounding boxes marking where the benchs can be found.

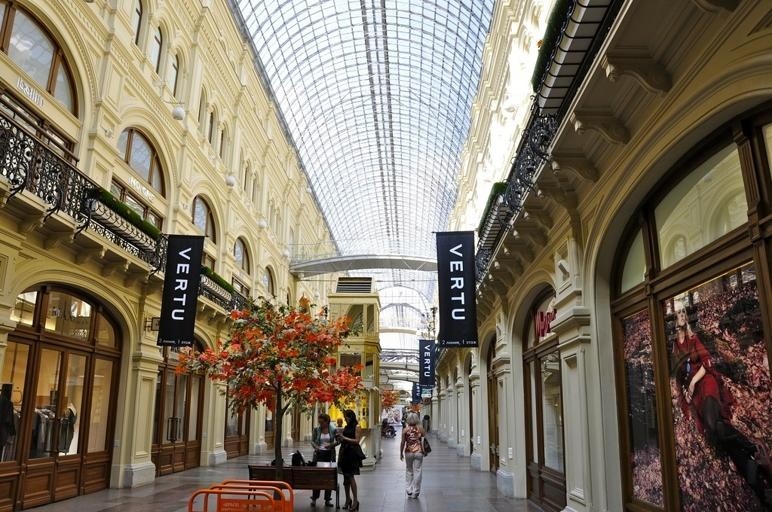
[246,463,341,510]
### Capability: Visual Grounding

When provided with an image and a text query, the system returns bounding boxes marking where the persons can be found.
[399,411,430,499]
[337,409,367,511]
[401,412,408,428]
[669,301,771,491]
[309,413,340,508]
[63,402,78,452]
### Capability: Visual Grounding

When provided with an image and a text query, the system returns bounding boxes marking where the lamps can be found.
[250,211,269,232]
[162,96,188,124]
[218,169,238,189]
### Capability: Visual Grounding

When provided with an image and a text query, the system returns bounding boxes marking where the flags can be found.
[156,233,205,349]
[434,231,479,348]
[411,339,436,406]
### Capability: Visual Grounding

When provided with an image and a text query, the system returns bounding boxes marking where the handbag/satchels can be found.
[421,436,431,456]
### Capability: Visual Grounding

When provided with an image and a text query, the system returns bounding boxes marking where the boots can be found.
[325,500,333,507]
[311,499,316,506]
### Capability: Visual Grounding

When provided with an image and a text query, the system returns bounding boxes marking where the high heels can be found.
[342,499,352,509]
[349,501,359,511]
